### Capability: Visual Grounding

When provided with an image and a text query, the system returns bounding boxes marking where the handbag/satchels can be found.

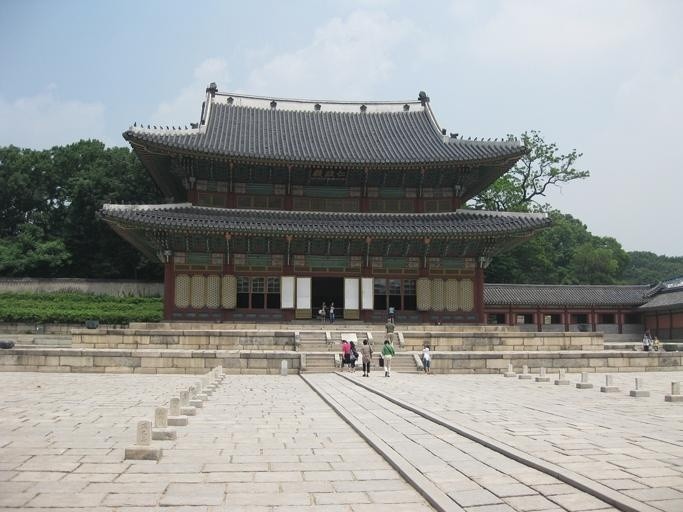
[379,357,384,367]
[350,349,357,361]
[352,348,358,357]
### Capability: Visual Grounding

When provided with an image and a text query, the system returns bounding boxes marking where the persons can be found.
[384,318,394,348]
[380,339,394,377]
[386,304,395,324]
[420,343,431,375]
[341,339,350,369]
[347,341,358,373]
[639,331,665,352]
[327,302,336,324]
[317,302,326,323]
[359,339,372,377]
